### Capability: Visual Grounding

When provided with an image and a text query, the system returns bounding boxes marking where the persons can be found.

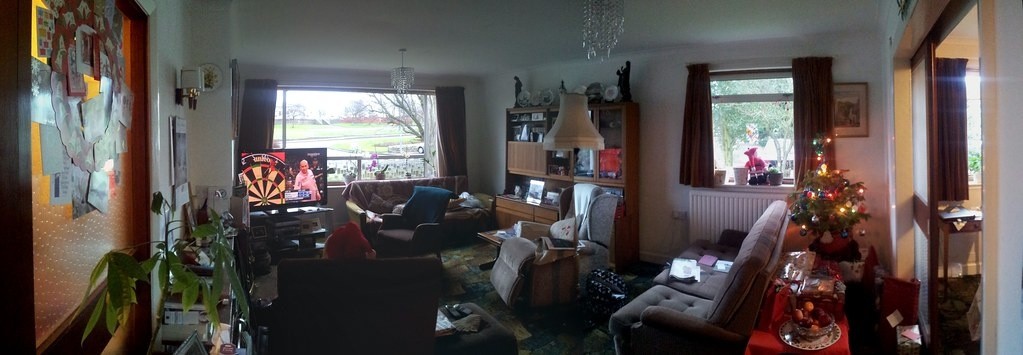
[286,156,324,200]
[616,61,632,100]
[514,76,523,103]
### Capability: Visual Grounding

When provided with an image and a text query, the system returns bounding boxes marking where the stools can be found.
[274,256,444,355]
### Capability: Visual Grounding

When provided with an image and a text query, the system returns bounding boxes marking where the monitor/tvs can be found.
[238,147,327,215]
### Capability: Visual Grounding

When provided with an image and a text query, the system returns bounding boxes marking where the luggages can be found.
[585,269,629,328]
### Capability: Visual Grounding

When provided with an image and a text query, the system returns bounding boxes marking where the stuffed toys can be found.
[744,148,766,185]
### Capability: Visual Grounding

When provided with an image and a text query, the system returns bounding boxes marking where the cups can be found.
[715,168,726,184]
[734,168,748,185]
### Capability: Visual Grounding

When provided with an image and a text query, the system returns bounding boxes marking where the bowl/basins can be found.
[792,311,835,342]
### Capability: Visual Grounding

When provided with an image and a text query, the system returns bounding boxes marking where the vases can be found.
[369,171,374,179]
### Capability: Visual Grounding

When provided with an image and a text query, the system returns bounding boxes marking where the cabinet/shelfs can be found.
[495,100,639,272]
[249,206,331,257]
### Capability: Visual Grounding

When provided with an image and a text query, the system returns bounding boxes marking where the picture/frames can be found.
[833,81,869,139]
[251,224,267,238]
[312,219,320,229]
[173,330,208,355]
[299,221,311,233]
[183,202,196,234]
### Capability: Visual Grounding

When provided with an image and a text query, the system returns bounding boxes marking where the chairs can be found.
[375,186,455,270]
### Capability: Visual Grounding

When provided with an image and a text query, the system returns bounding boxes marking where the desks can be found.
[433,301,517,355]
[744,251,851,355]
[145,238,241,355]
[939,209,986,302]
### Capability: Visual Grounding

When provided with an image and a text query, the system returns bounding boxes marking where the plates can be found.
[516,82,620,107]
[779,320,841,350]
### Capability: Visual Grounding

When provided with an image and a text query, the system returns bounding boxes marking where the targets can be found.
[240,153,287,206]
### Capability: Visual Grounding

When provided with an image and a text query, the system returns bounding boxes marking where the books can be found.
[669,258,701,282]
[698,255,719,266]
[532,236,586,251]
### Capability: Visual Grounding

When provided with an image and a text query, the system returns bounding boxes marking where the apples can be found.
[790,301,832,330]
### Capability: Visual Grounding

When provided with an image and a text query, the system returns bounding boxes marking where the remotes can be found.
[445,304,473,318]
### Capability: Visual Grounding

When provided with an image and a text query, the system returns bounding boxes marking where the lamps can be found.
[582,0,625,61]
[542,93,605,251]
[176,66,202,111]
[391,47,416,96]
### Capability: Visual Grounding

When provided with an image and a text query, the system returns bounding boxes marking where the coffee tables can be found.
[478,223,594,305]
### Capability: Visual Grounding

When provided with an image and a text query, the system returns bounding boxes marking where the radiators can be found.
[689,190,794,244]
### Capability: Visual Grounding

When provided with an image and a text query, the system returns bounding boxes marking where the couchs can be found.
[344,175,487,241]
[608,201,792,354]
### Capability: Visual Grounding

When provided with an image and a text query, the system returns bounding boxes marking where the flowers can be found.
[367,153,379,173]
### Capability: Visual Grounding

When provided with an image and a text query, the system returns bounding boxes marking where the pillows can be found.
[369,194,393,215]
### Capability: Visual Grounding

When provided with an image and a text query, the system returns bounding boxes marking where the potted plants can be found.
[343,170,357,187]
[770,166,782,185]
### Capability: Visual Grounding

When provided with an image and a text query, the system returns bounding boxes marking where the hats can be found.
[323,221,376,259]
